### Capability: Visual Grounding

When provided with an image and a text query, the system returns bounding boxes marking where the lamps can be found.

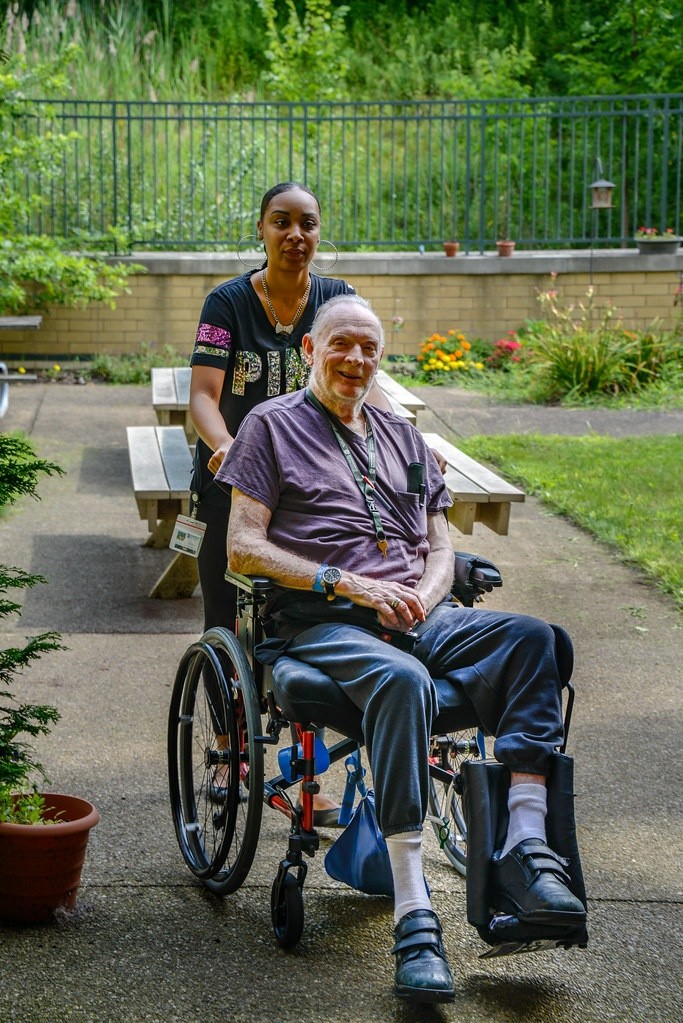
[587,176,616,210]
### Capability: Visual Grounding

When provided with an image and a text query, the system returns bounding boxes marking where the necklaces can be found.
[259,264,310,334]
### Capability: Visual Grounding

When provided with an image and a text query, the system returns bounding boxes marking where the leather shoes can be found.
[391,910,456,1001]
[491,839,588,924]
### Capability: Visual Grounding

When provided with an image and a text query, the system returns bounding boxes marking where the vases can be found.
[495,240,516,256]
[637,236,683,256]
[443,241,460,257]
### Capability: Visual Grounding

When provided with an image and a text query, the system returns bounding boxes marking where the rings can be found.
[391,599,401,609]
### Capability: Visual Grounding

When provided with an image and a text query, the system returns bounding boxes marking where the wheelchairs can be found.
[168,552,588,958]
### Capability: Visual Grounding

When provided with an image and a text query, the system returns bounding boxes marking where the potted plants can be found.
[0,791,100,927]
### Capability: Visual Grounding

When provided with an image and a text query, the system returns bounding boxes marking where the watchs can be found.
[323,565,341,601]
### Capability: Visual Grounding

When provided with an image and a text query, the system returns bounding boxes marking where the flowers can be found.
[636,227,674,241]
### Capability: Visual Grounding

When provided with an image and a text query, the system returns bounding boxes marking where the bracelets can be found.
[312,563,331,593]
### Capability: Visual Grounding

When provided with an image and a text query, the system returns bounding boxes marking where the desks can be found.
[0,312,45,425]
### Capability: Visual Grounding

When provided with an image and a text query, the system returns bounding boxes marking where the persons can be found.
[186,181,448,825]
[213,297,590,1001]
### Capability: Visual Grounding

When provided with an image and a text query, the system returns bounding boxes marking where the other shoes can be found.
[207,765,248,802]
[293,796,350,826]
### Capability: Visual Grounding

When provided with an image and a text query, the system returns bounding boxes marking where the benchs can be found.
[151,365,193,435]
[373,370,426,427]
[125,425,195,530]
[415,431,527,537]
[0,373,38,382]
